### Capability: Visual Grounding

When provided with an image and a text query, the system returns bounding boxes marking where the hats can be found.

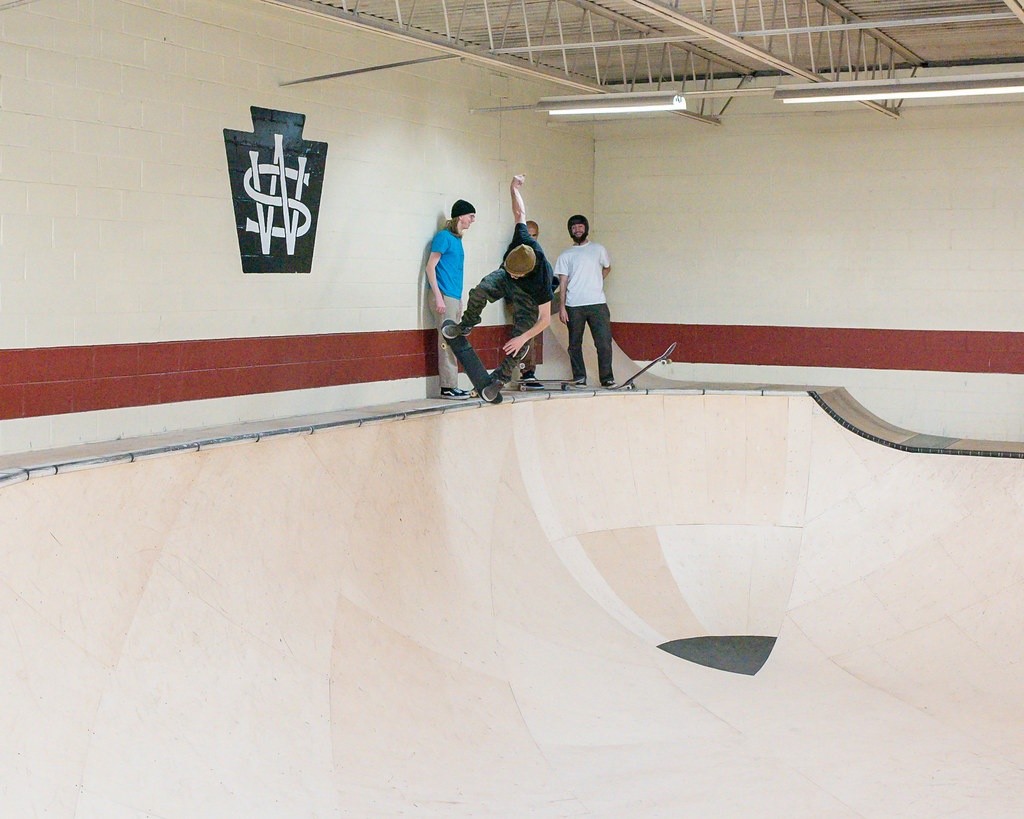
[450,199,477,218]
[503,244,536,275]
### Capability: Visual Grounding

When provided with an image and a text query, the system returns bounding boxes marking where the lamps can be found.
[772,72,1024,104]
[534,94,688,116]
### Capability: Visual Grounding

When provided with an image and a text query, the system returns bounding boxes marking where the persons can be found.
[552,215,620,389]
[504,220,555,389]
[442,172,556,401]
[424,198,478,400]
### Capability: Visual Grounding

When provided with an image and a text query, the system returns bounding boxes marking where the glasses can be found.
[528,232,538,238]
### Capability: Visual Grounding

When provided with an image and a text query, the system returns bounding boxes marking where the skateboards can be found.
[510,374,585,391]
[440,318,504,405]
[607,341,677,391]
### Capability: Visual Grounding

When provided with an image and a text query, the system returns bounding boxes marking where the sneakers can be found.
[601,380,617,389]
[482,369,506,402]
[521,383,544,390]
[523,370,535,381]
[441,321,472,339]
[572,380,587,388]
[441,385,472,400]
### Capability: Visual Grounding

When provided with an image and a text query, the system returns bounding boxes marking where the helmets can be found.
[566,215,590,242]
